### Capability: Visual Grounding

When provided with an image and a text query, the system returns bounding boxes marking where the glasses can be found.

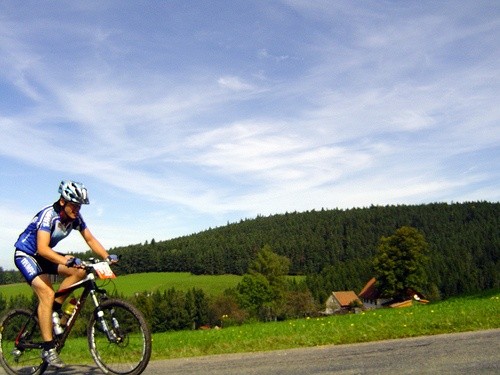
[67,202,81,209]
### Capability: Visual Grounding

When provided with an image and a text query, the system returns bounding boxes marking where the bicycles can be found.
[0,250,152,375]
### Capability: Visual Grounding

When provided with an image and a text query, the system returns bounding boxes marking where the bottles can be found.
[52,310,63,336]
[60,299,76,326]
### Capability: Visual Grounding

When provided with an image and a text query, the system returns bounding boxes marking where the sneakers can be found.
[42,347,65,367]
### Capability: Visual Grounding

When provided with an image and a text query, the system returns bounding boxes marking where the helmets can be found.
[58,180,90,204]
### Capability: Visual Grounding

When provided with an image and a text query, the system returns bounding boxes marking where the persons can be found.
[13,180,118,368]
